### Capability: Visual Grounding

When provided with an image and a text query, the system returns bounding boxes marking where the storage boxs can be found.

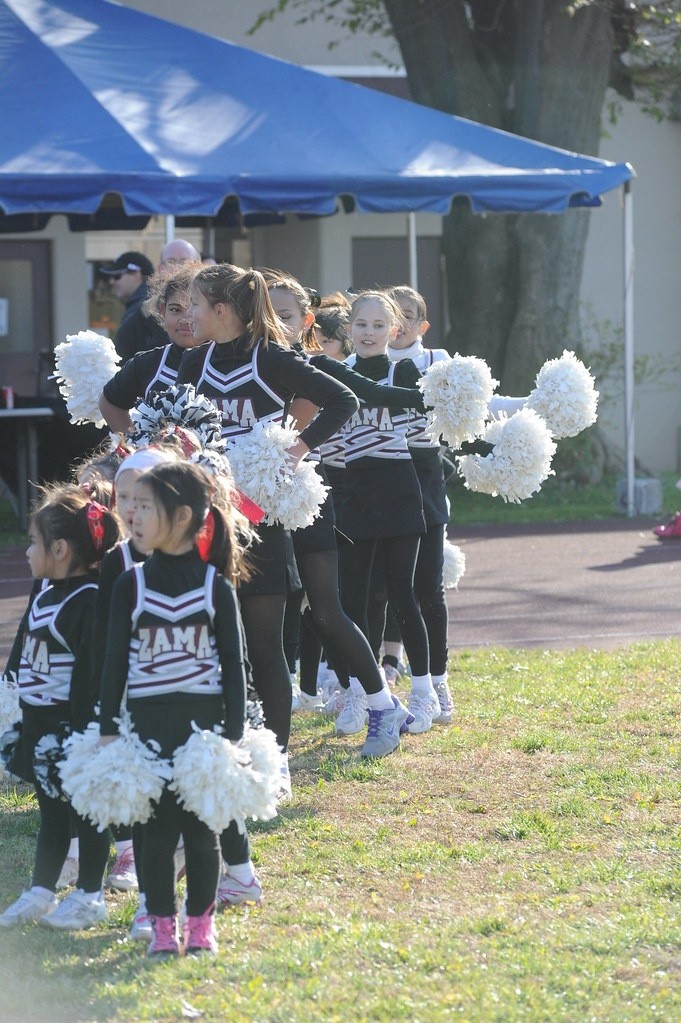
[615,477,664,515]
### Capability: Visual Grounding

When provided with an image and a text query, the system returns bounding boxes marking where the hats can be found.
[98,252,154,277]
[302,285,321,307]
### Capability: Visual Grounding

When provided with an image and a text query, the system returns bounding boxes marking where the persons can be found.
[0,239,600,958]
[652,511,681,539]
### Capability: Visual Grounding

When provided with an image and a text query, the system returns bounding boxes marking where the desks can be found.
[0,407,54,530]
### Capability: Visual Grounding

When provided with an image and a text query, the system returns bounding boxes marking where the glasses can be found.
[105,273,134,281]
[159,260,189,268]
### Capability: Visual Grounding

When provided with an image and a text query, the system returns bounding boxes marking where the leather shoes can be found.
[653,510,681,540]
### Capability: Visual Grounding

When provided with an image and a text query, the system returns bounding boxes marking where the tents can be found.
[0,0,637,523]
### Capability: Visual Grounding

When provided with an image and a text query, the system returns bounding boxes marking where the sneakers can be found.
[334,687,370,735]
[174,846,187,884]
[361,694,416,761]
[130,892,153,940]
[55,856,79,889]
[378,661,412,688]
[216,873,262,910]
[146,912,180,962]
[41,888,107,931]
[406,688,442,734]
[0,885,59,930]
[182,900,219,959]
[278,754,292,804]
[432,679,453,725]
[290,673,346,718]
[104,846,139,889]
